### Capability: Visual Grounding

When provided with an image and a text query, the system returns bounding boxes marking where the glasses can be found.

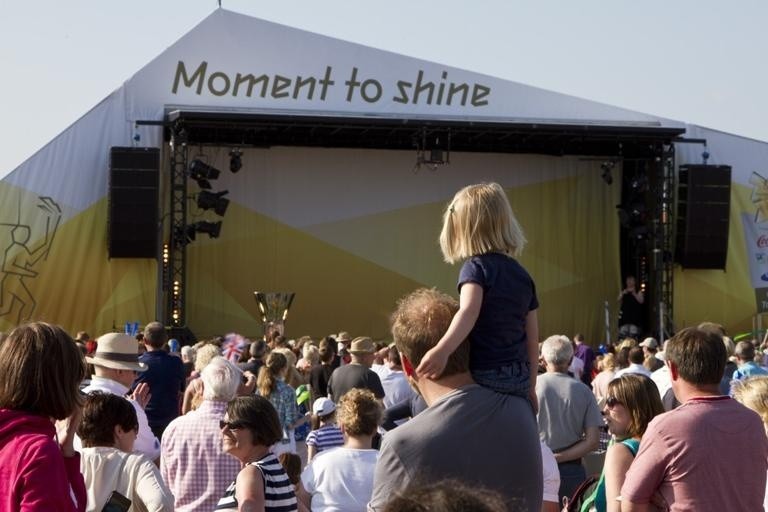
[606,397,625,407]
[219,419,256,430]
[126,423,139,433]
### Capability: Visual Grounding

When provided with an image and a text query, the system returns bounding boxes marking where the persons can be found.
[365,288,545,512]
[134,332,148,357]
[181,344,223,412]
[730,372,768,438]
[696,321,728,345]
[255,353,312,448]
[75,391,174,512]
[640,335,666,374]
[160,356,247,512]
[598,342,617,358]
[71,332,162,467]
[306,396,344,469]
[128,320,190,440]
[376,345,419,447]
[265,330,281,349]
[301,343,320,388]
[618,336,639,348]
[617,276,646,344]
[83,340,100,381]
[720,333,738,396]
[320,336,345,370]
[0,321,88,512]
[72,328,89,361]
[309,341,336,398]
[214,395,300,512]
[178,344,196,391]
[581,373,667,512]
[616,347,653,380]
[573,333,596,386]
[618,326,768,512]
[592,353,620,416]
[240,339,268,393]
[414,183,539,417]
[336,332,353,366]
[295,388,381,512]
[326,336,385,409]
[273,336,294,386]
[536,335,601,512]
[168,339,181,359]
[732,340,768,384]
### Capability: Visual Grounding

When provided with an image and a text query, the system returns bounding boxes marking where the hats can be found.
[639,337,658,350]
[312,397,336,417]
[336,332,351,343]
[85,333,149,372]
[346,337,376,354]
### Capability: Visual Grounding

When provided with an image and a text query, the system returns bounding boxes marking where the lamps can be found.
[187,220,222,239]
[413,127,452,172]
[228,148,244,173]
[187,189,231,217]
[598,155,620,185]
[189,147,222,190]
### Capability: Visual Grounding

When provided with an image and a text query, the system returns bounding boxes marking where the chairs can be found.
[568,474,599,512]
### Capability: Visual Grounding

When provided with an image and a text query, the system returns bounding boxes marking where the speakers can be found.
[678,163,731,271]
[107,145,158,262]
